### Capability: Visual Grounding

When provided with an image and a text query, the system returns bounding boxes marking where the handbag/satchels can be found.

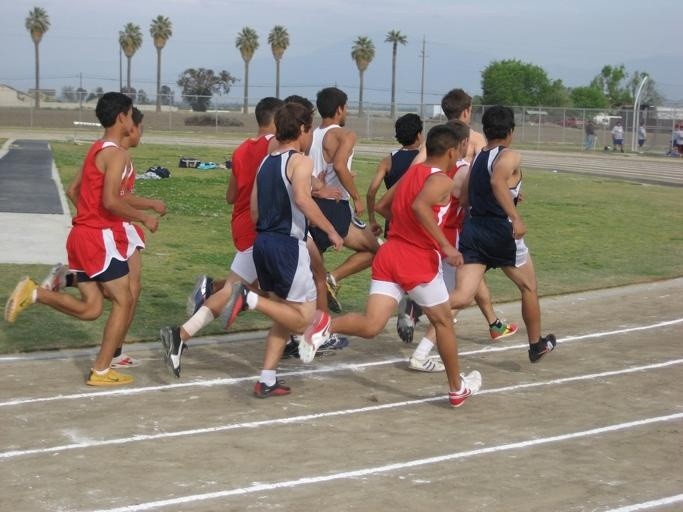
[178,156,232,171]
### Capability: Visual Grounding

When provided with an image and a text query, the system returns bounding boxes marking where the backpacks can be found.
[145,165,171,180]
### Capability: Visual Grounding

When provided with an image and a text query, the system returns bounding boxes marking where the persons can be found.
[611,122,624,152]
[584,121,594,149]
[668,124,679,156]
[675,125,683,155]
[5,93,159,385]
[638,122,646,152]
[157,87,565,405]
[38,108,168,371]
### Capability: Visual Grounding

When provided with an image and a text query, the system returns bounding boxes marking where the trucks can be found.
[592,115,622,129]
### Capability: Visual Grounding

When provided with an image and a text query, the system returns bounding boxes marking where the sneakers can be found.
[396,296,421,345]
[280,270,351,366]
[528,334,557,364]
[448,370,483,408]
[2,260,74,324]
[186,273,252,331]
[159,324,189,381]
[253,378,292,399]
[407,356,446,372]
[488,323,519,341]
[86,352,144,386]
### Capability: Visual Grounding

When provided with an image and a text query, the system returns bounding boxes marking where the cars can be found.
[560,118,587,129]
